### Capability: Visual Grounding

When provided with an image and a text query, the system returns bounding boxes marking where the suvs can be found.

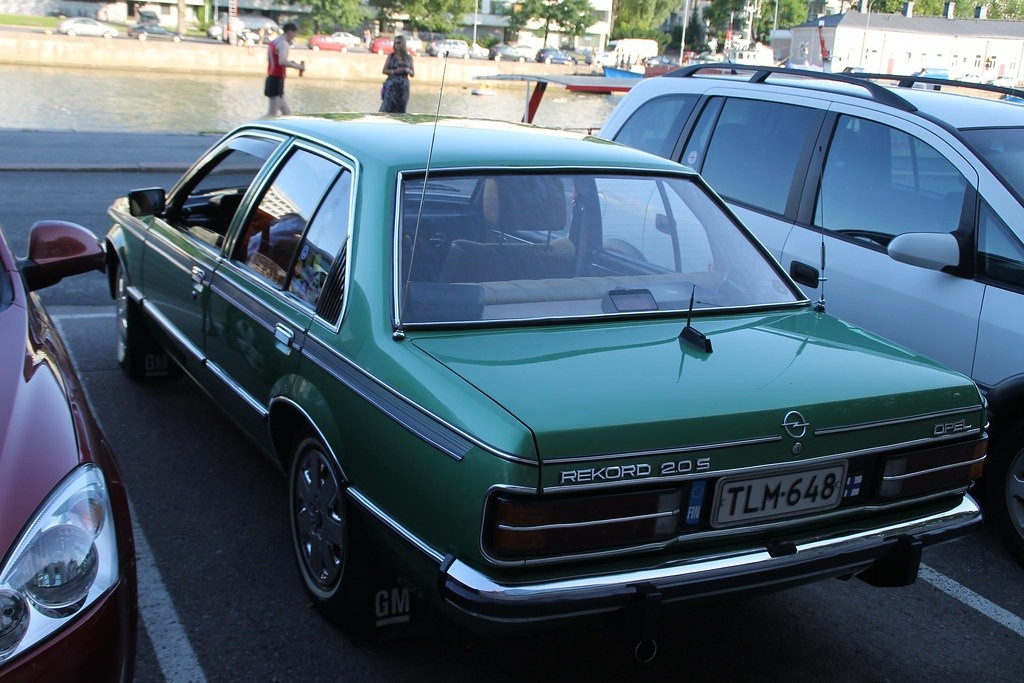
[594,63,1023,549]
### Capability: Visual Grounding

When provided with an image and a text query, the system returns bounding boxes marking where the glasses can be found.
[395,42,402,44]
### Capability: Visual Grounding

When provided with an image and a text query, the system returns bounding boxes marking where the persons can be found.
[379,35,414,113]
[364,30,372,49]
[264,22,305,116]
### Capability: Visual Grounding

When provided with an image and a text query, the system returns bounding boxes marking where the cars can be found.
[105,113,989,639]
[400,35,422,49]
[0,218,137,683]
[331,32,361,46]
[488,43,533,63]
[60,16,119,42]
[536,48,577,65]
[308,35,348,53]
[371,36,418,58]
[127,23,184,45]
[585,54,612,65]
[606,38,659,61]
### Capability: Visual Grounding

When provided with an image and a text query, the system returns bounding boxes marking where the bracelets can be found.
[390,70,394,75]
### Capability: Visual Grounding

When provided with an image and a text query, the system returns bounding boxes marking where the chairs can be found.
[712,124,923,235]
[441,175,577,283]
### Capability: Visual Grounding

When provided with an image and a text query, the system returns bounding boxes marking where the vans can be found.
[430,38,469,58]
